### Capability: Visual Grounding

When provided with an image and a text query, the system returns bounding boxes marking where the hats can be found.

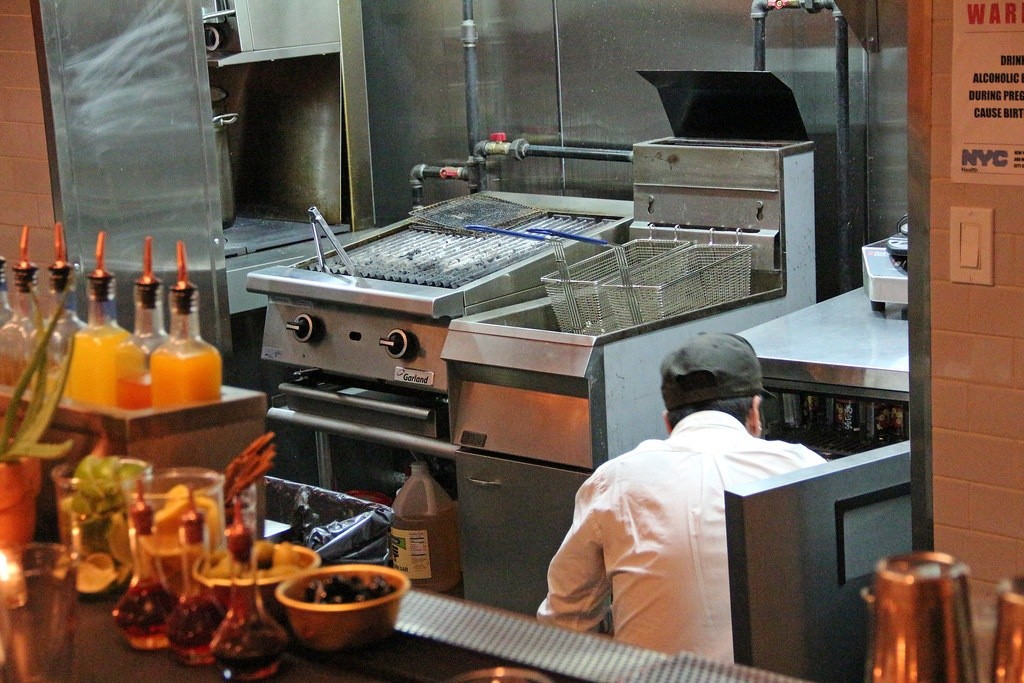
[661,331,776,410]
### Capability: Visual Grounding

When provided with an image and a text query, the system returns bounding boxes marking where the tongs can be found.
[308,206,362,278]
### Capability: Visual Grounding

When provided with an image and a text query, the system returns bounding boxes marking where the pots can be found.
[209,84,240,229]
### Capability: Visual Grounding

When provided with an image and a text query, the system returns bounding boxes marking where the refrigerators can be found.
[733,285,914,683]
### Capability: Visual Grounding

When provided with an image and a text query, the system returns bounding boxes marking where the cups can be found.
[124,467,225,594]
[1,543,79,683]
[50,455,154,598]
[449,667,551,683]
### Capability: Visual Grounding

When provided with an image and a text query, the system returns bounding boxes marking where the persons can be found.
[534,328,833,661]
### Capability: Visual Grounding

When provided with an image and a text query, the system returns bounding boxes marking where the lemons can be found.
[67,455,146,590]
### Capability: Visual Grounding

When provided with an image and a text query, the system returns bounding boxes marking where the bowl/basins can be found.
[276,563,411,650]
[193,543,322,611]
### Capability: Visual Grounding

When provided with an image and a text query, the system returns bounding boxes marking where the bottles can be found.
[115,236,169,409]
[166,485,227,664]
[390,460,461,591]
[67,230,133,408]
[861,551,979,683]
[0,225,46,389]
[150,239,223,407]
[990,576,1024,683]
[109,478,181,651]
[30,221,89,397]
[207,493,289,680]
